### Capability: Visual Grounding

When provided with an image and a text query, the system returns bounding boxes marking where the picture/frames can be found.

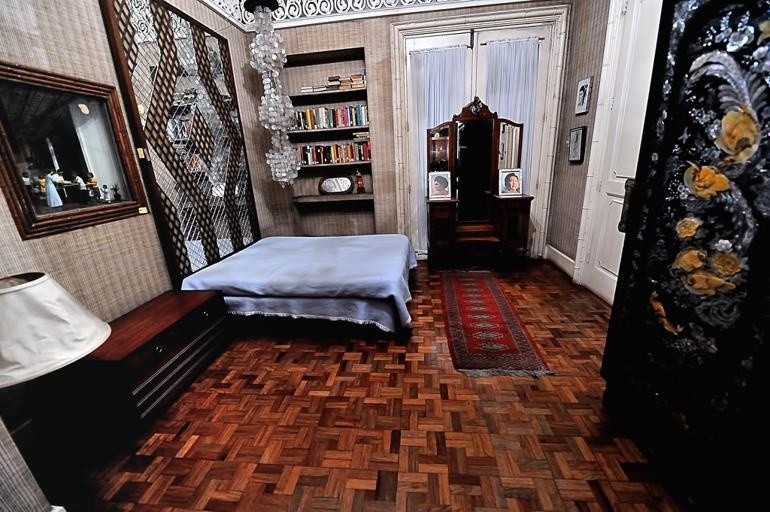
[1,59,151,242]
[568,125,587,163]
[575,76,594,116]
[498,168,523,195]
[429,171,452,200]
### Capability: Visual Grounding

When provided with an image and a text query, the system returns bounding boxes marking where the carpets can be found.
[440,267,553,377]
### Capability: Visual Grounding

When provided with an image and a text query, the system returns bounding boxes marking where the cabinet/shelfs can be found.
[64,291,234,439]
[284,48,375,236]
[425,95,534,277]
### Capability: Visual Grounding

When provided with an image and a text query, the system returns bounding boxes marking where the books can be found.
[282,72,372,167]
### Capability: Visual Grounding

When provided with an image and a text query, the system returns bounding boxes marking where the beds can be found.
[180,234,418,352]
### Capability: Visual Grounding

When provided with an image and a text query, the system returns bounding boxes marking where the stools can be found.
[453,236,502,271]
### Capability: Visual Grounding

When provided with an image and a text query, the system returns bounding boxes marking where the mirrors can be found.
[318,176,354,194]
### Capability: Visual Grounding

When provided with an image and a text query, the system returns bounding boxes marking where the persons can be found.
[503,173,520,193]
[431,175,449,196]
[577,85,585,107]
[19,168,112,211]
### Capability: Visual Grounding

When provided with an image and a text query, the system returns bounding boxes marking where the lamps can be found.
[244,0,302,189]
[0,271,111,388]
